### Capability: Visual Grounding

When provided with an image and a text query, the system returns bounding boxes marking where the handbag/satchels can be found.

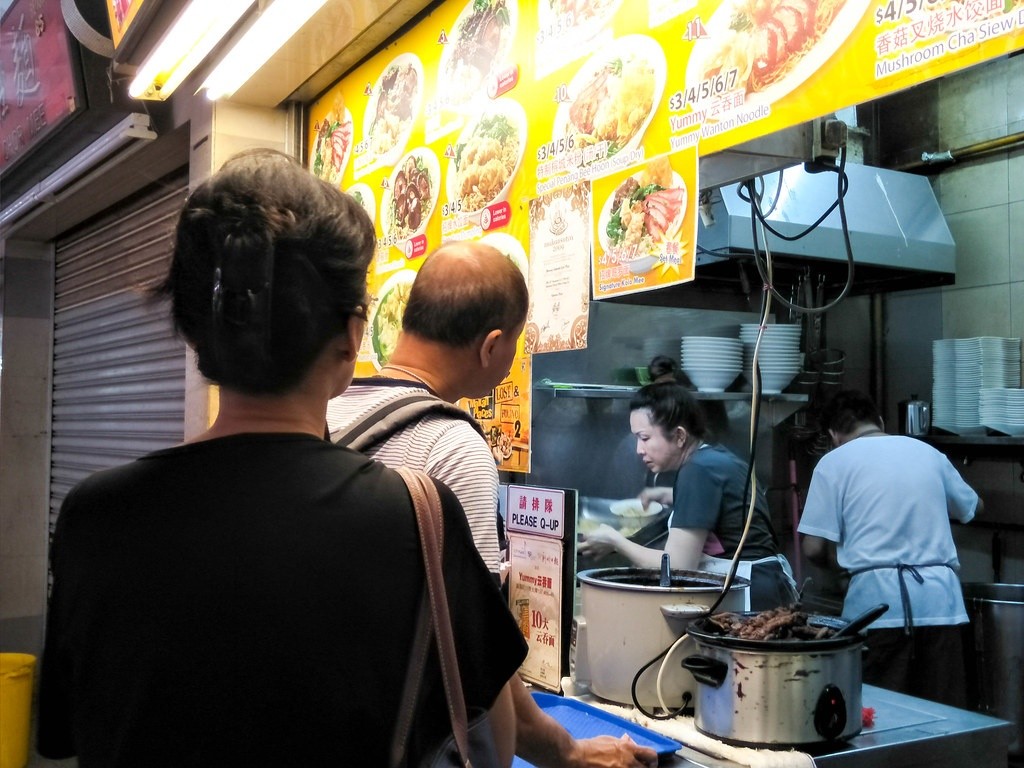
[433,711,498,768]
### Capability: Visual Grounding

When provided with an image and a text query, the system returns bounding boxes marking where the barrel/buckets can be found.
[0,653,38,768]
[961,582,1024,753]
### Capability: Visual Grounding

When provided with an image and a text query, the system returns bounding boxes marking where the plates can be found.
[539,0,623,51]
[480,232,528,287]
[686,0,871,120]
[310,109,353,189]
[344,184,376,225]
[932,336,1024,438]
[436,0,518,106]
[364,55,425,166]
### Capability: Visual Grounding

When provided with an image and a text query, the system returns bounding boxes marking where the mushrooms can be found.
[393,156,431,228]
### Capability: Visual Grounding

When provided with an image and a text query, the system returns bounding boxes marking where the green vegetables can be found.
[606,184,664,242]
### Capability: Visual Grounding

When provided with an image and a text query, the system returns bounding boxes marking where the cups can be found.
[898,394,930,436]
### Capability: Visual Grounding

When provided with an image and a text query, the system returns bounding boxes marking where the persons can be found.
[577,355,803,612]
[33,147,517,768]
[797,389,985,711]
[326,240,658,768]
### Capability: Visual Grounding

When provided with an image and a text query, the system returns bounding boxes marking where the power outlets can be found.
[811,111,842,161]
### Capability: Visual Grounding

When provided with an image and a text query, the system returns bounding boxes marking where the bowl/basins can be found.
[681,324,801,393]
[553,34,667,171]
[368,269,417,372]
[380,148,440,243]
[446,99,527,217]
[599,170,688,274]
[609,498,663,528]
[634,366,675,386]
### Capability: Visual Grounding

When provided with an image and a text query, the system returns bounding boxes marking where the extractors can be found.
[695,159,958,310]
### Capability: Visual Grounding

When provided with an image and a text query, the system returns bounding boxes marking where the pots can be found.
[681,611,870,750]
[571,567,751,715]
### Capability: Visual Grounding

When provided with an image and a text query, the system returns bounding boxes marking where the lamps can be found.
[0,112,157,241]
